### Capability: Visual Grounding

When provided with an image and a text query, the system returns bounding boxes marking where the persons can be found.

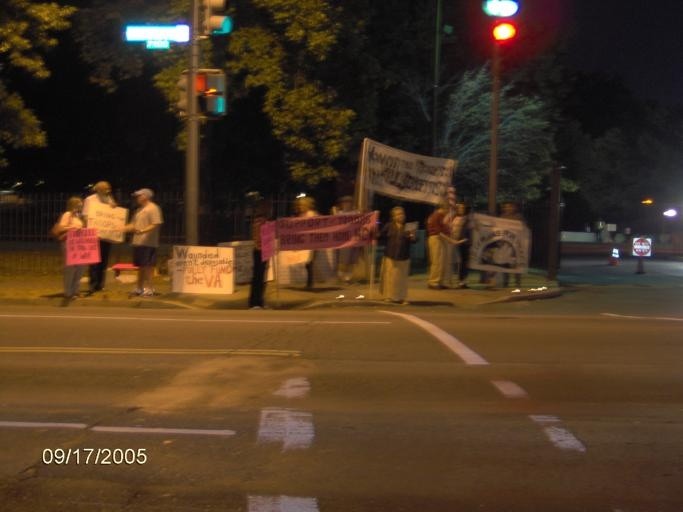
[51,196,87,299]
[82,180,117,292]
[114,187,165,295]
[249,196,527,309]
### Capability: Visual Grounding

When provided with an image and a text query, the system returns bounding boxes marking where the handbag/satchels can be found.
[52,224,68,239]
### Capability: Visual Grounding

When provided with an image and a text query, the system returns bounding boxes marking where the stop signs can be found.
[633,237,652,257]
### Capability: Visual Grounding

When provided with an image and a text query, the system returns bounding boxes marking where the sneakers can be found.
[130,288,153,297]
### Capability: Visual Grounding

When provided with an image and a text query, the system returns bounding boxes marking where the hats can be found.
[132,188,152,198]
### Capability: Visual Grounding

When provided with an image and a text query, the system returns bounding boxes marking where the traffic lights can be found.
[196,70,228,116]
[482,0,518,44]
[201,0,232,37]
